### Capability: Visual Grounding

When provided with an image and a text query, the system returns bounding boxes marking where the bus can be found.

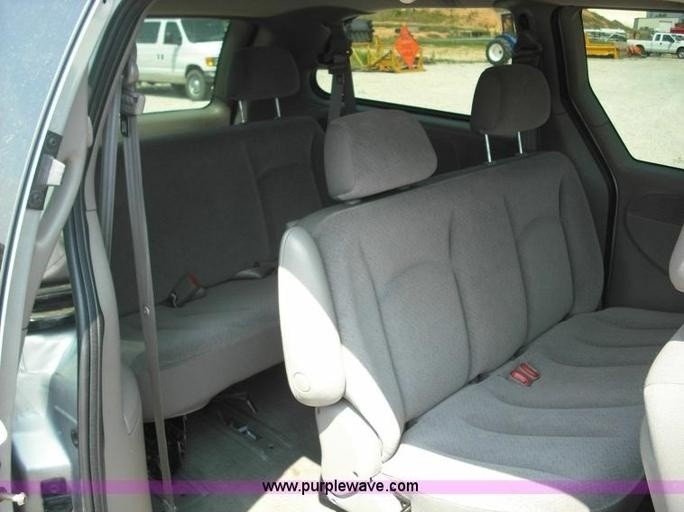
[584,27,627,43]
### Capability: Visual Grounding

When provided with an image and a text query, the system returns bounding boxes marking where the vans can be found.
[134,15,229,100]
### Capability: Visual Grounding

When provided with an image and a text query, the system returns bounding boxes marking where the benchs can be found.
[276,64,683,512]
[95,44,335,440]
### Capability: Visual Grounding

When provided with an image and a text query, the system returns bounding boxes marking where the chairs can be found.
[639,229,683,512]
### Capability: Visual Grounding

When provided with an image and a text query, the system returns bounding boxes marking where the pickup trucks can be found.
[624,33,684,58]
